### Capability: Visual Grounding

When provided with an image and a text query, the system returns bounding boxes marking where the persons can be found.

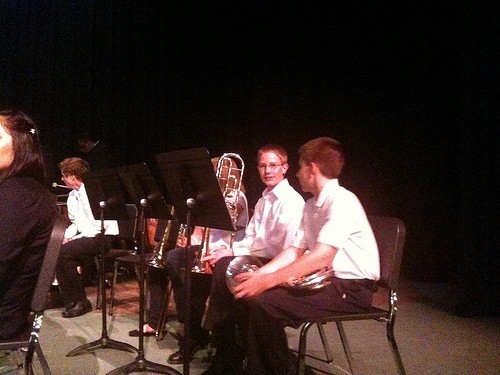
[129,152,247,337]
[55,155,125,318]
[76,121,125,209]
[232,137,383,375]
[167,145,306,375]
[1,113,63,341]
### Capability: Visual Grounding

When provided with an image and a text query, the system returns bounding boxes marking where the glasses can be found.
[258,163,282,168]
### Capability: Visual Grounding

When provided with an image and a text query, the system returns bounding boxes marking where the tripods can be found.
[65,147,182,375]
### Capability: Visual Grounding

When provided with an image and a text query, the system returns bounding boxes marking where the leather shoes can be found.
[168,333,211,364]
[62,301,92,318]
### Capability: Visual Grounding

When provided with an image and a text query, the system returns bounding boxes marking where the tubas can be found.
[143,205,186,269]
[186,152,246,275]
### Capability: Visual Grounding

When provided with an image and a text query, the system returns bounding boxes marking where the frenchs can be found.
[224,254,335,300]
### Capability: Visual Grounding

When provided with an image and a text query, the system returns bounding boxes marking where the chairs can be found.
[296,215,405,375]
[93,204,254,342]
[0,216,71,375]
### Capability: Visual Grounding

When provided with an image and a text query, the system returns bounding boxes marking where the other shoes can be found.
[129,324,160,336]
